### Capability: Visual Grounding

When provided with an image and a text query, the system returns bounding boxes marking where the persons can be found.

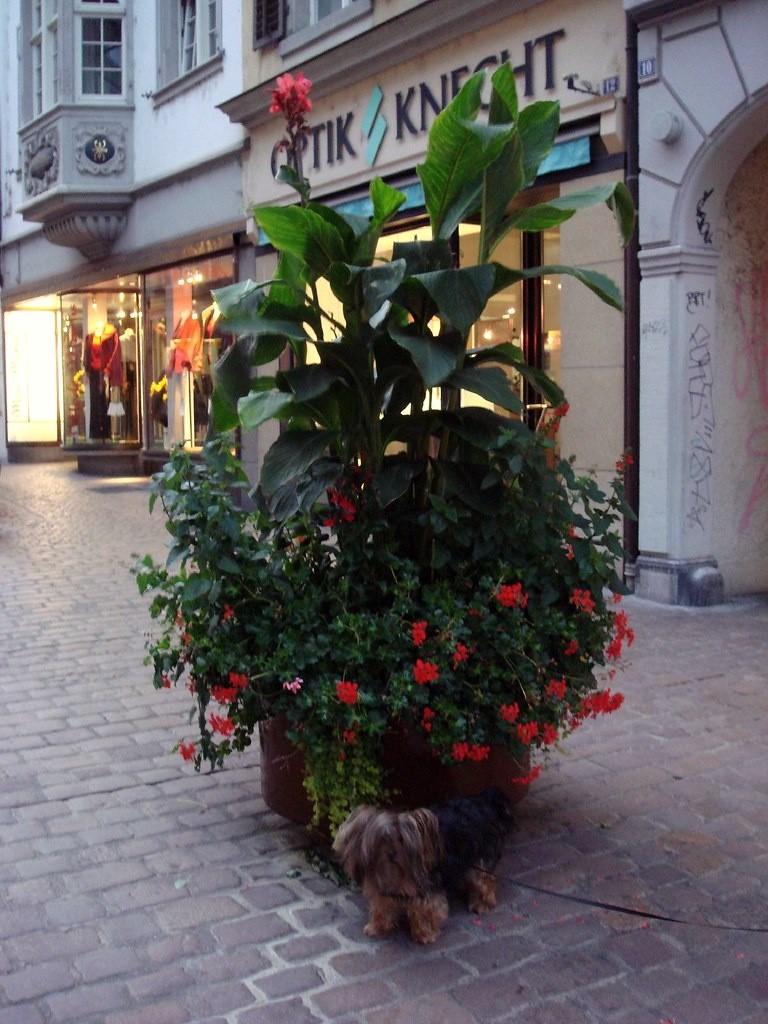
[84,324,121,439]
[119,327,136,440]
[202,302,225,395]
[166,306,201,452]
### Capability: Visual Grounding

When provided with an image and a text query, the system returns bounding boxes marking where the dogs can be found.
[332,789,514,944]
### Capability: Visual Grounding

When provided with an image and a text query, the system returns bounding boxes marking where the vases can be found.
[260,712,531,823]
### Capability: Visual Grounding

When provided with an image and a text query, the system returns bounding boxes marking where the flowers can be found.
[128,64,634,835]
